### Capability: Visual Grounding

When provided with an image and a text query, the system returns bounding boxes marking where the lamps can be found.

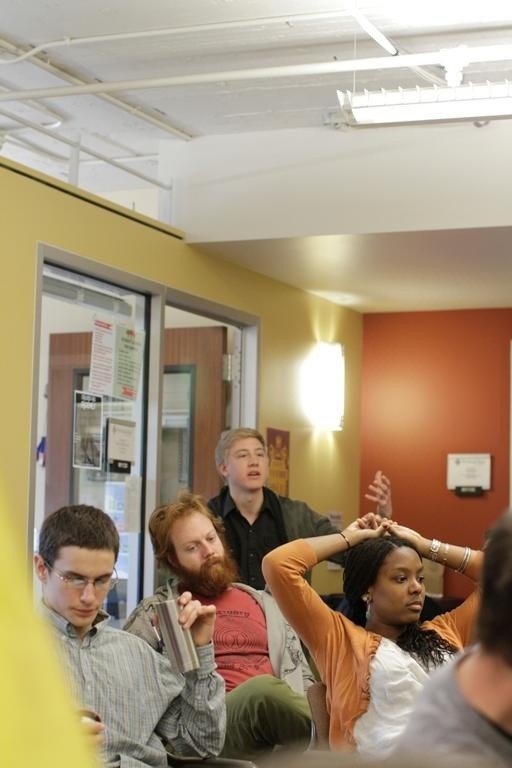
[344,0,398,56]
[337,62,512,130]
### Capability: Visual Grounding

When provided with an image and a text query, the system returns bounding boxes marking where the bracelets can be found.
[455,546,471,573]
[443,543,449,565]
[427,539,442,562]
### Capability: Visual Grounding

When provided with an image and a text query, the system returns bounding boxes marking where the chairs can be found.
[165,749,260,768]
[307,683,331,752]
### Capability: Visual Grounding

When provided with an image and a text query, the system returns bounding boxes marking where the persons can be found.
[386,508,512,768]
[262,511,485,762]
[29,505,227,768]
[207,428,393,597]
[123,488,319,761]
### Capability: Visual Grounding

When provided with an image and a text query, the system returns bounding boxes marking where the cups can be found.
[154,599,201,673]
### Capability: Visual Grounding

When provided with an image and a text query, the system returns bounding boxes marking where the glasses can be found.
[44,560,119,591]
